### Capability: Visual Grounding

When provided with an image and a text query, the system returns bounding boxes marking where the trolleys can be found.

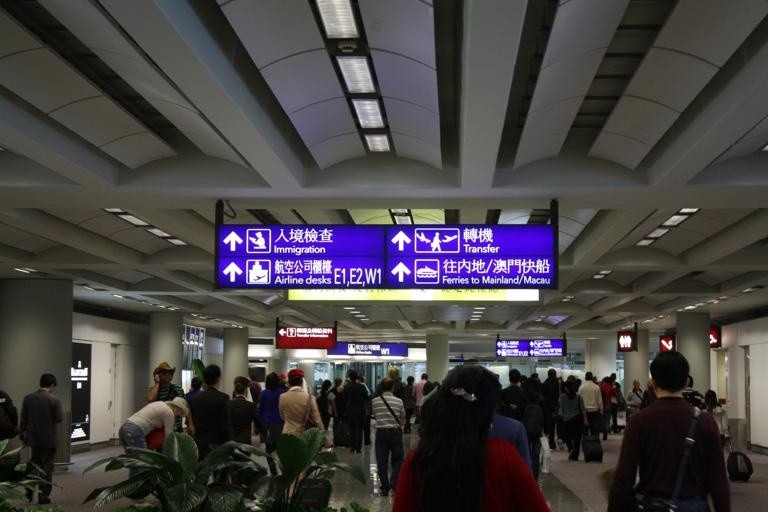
[626,403,639,423]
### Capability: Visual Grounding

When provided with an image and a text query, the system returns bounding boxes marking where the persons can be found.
[372,378,406,497]
[119,362,334,510]
[608,353,732,510]
[315,368,440,454]
[542,369,644,461]
[392,365,549,510]
[19,373,63,504]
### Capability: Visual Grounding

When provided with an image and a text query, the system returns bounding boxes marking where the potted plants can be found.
[292,442,370,509]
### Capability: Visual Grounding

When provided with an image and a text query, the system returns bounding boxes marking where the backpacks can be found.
[521,395,544,433]
[316,390,330,414]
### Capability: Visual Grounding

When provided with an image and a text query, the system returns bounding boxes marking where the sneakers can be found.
[548,436,579,460]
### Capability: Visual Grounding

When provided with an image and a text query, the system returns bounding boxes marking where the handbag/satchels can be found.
[630,487,678,512]
[304,407,317,429]
[19,430,36,446]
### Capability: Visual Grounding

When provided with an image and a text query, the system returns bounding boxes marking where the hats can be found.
[153,362,174,376]
[288,370,305,375]
[165,397,189,417]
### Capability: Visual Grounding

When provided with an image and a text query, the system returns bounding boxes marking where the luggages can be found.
[727,437,753,482]
[293,444,332,509]
[333,416,351,446]
[583,426,602,462]
